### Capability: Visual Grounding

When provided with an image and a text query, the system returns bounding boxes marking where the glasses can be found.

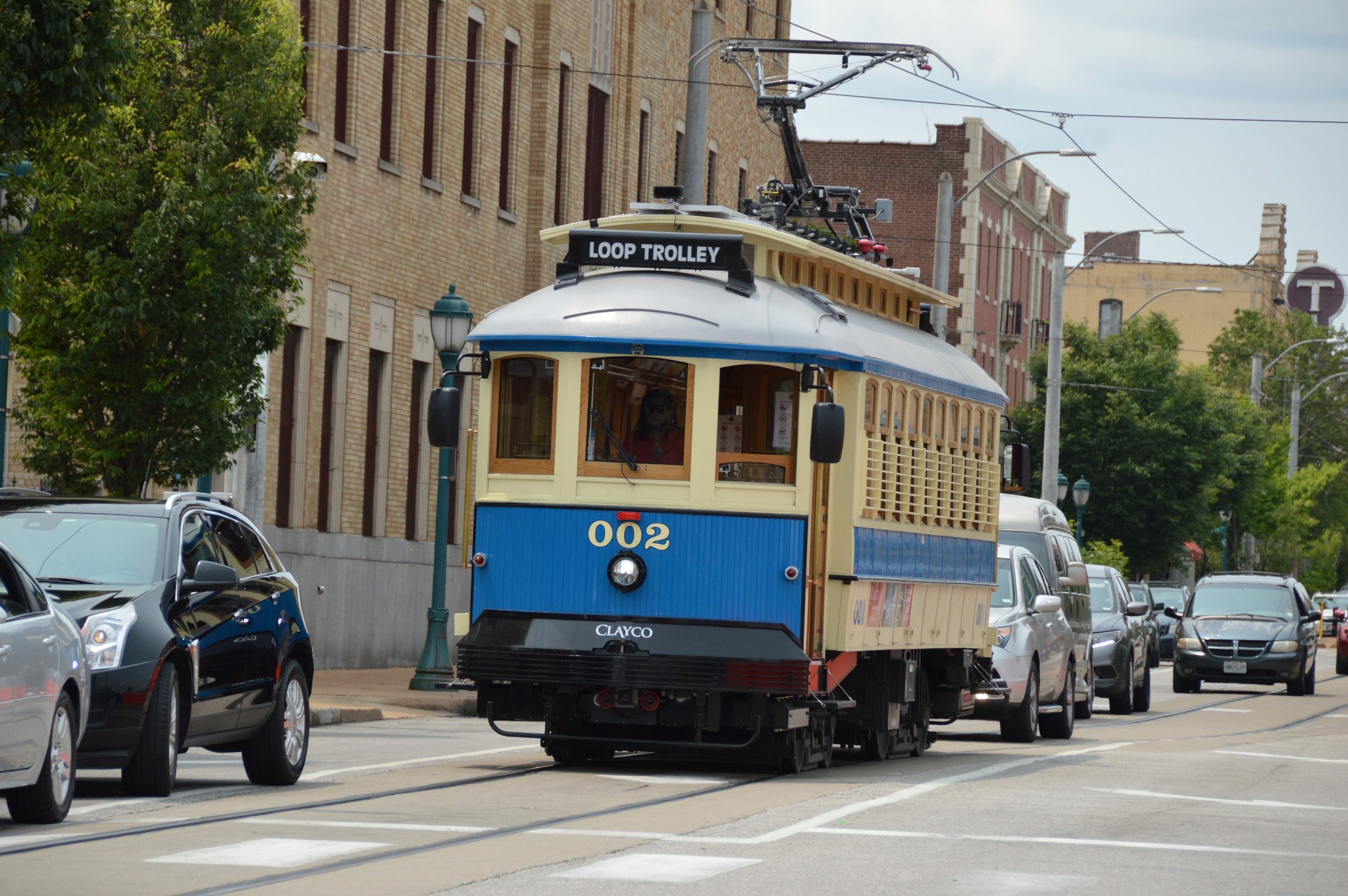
[644,404,665,415]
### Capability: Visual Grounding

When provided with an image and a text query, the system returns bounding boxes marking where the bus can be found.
[466,36,1033,772]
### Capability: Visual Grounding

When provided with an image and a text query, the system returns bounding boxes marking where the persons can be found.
[618,387,685,465]
[1140,573,1151,590]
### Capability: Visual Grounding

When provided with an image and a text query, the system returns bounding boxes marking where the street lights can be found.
[932,146,1098,350]
[1056,468,1090,551]
[406,284,469,689]
[1042,227,1184,514]
[1243,338,1346,573]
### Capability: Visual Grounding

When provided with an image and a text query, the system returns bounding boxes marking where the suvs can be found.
[1145,582,1193,650]
[1164,573,1324,696]
[0,485,316,797]
[1125,579,1163,666]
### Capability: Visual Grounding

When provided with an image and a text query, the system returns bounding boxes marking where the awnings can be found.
[1183,540,1205,563]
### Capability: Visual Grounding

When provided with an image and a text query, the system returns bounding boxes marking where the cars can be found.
[1333,609,1348,673]
[973,544,1076,745]
[0,541,93,823]
[1310,591,1348,636]
[1086,565,1151,715]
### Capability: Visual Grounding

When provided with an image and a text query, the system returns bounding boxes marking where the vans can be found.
[997,491,1098,718]
